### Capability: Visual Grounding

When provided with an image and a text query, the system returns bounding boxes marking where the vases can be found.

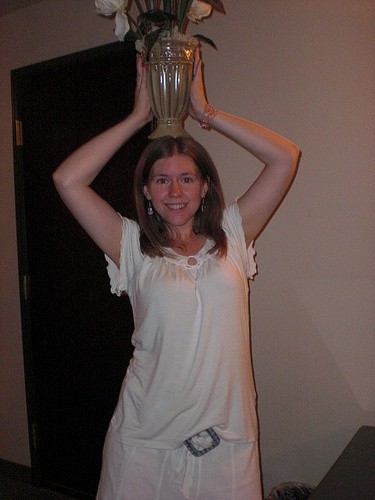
[141,38,199,139]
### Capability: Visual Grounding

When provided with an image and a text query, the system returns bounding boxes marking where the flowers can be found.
[93,0,227,60]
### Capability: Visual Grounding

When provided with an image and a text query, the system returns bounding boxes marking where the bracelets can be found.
[197,102,216,131]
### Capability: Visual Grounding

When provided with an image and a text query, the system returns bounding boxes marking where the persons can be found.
[50,41,301,500]
